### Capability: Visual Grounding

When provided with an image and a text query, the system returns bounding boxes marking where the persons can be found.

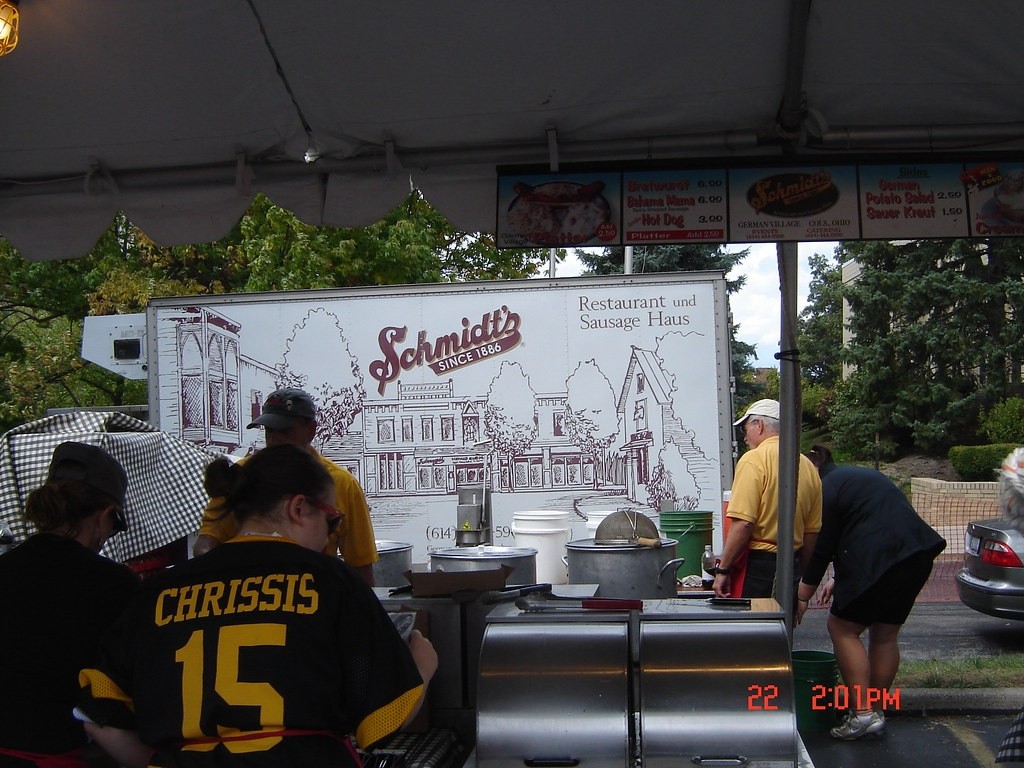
[193,387,378,584]
[793,445,947,743]
[712,399,822,624]
[0,442,437,768]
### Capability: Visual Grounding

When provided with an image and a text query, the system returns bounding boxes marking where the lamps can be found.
[0,0,20,57]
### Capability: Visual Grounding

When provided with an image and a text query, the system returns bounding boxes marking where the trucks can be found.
[81,269,738,569]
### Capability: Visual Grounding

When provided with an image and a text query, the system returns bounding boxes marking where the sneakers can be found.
[831,710,884,739]
[855,713,888,739]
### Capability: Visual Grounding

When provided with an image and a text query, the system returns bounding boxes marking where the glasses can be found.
[278,492,345,537]
[740,419,759,433]
[109,506,122,538]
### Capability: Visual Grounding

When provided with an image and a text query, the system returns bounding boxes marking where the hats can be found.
[48,441,128,533]
[246,388,316,430]
[733,399,781,425]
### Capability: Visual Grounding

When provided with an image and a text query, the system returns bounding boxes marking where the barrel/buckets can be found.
[428,544,538,584]
[658,511,714,579]
[511,510,570,585]
[337,538,414,587]
[790,650,840,733]
[585,511,644,540]
[561,536,686,599]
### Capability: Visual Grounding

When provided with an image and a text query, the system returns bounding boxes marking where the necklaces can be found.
[244,532,282,537]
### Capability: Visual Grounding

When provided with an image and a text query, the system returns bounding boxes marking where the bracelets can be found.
[798,599,807,601]
[832,577,835,579]
[716,563,729,575]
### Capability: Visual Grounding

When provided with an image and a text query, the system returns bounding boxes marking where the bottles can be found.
[700,544,716,590]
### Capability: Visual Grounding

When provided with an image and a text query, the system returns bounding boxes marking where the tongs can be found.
[453,580,550,605]
[515,591,644,615]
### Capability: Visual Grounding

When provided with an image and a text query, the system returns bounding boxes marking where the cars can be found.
[954,512,1024,622]
